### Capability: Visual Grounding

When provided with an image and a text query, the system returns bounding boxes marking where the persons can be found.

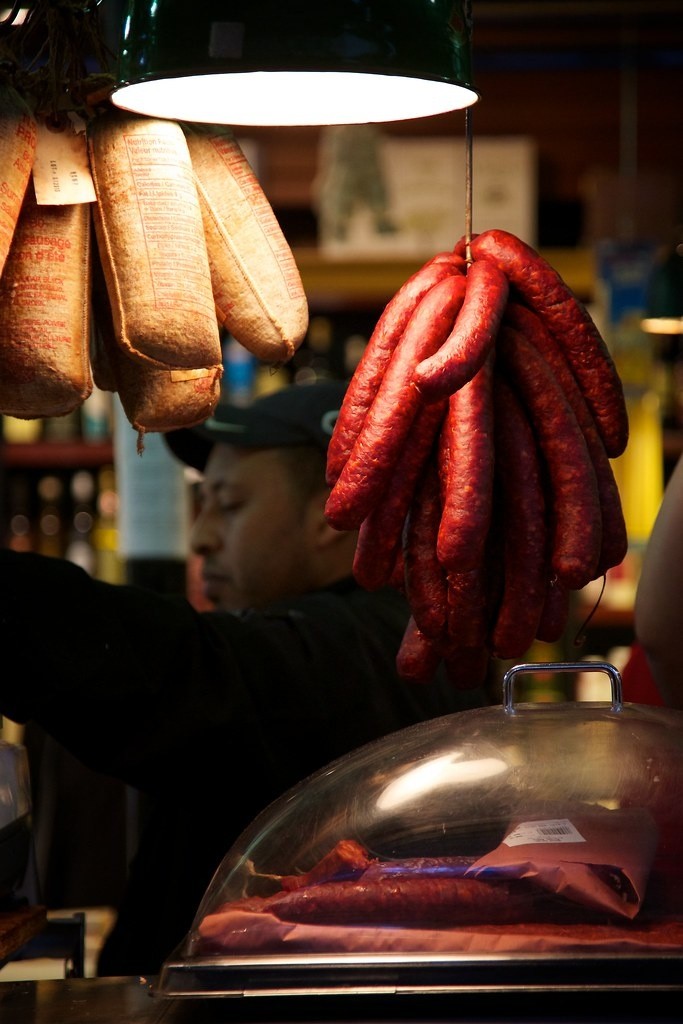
[2,376,511,981]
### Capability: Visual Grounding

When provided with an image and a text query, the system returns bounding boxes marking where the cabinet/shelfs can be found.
[3,23,683,627]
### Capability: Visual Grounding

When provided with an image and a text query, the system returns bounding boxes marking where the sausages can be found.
[325,235,629,690]
[197,838,683,958]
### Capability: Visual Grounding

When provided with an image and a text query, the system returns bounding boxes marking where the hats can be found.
[165,376,355,468]
